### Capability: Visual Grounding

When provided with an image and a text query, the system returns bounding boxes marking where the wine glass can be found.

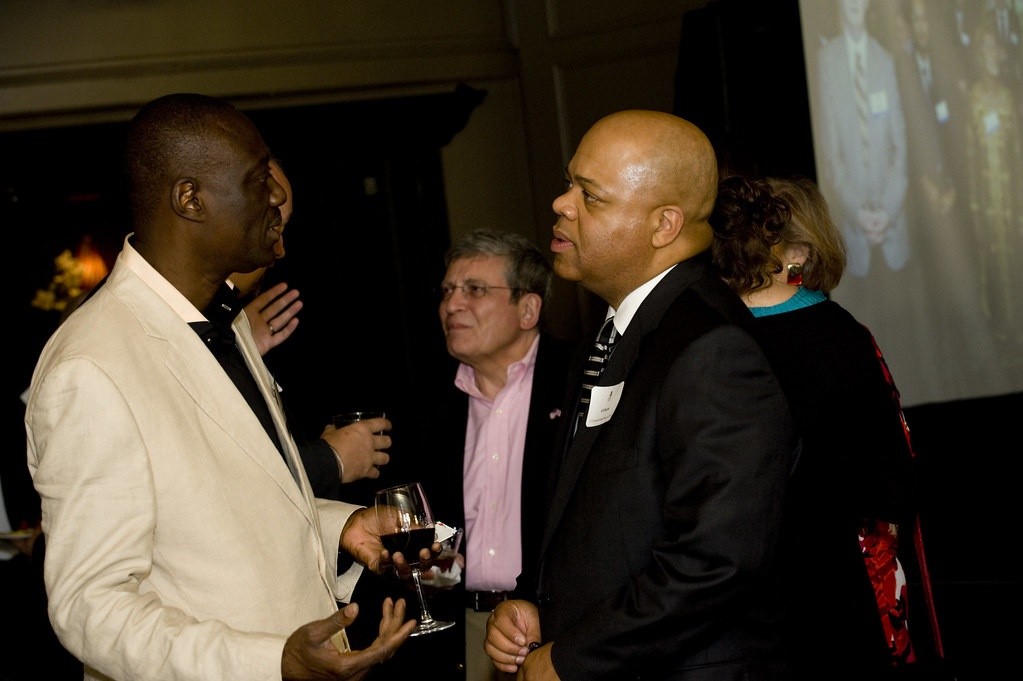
[374,481,457,636]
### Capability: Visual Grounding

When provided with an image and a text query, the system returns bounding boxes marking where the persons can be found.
[482,110,894,681]
[25,89,443,681]
[395,229,595,681]
[713,166,920,669]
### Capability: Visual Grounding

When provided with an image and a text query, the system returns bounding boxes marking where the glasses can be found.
[438,280,533,299]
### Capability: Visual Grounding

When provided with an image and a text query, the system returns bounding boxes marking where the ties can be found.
[573,316,620,436]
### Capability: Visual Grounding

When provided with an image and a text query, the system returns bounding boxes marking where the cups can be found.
[332,411,385,470]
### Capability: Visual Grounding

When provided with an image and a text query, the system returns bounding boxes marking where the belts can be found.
[466,590,520,613]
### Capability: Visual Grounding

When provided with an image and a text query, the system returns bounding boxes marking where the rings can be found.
[268,323,274,334]
